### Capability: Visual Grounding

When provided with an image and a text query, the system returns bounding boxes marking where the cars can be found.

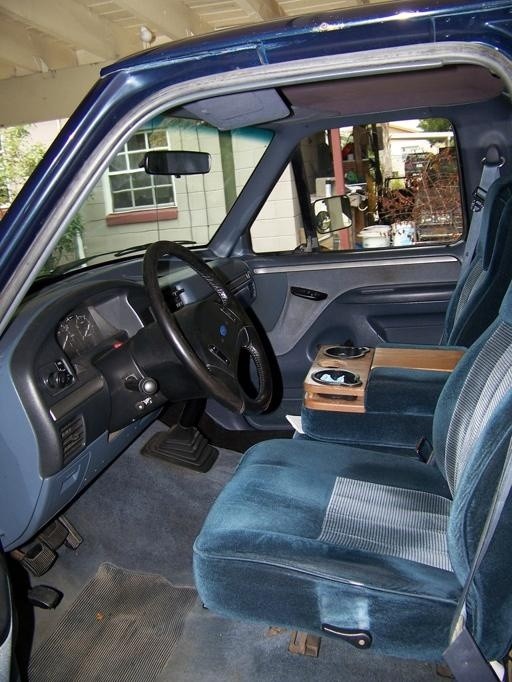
[0,0,512,680]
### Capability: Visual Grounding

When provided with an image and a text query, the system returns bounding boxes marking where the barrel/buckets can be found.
[391,220,415,247]
[325,184,331,197]
[357,224,391,248]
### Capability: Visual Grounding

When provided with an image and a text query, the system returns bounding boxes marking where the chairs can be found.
[300,176,511,445]
[191,267,512,667]
